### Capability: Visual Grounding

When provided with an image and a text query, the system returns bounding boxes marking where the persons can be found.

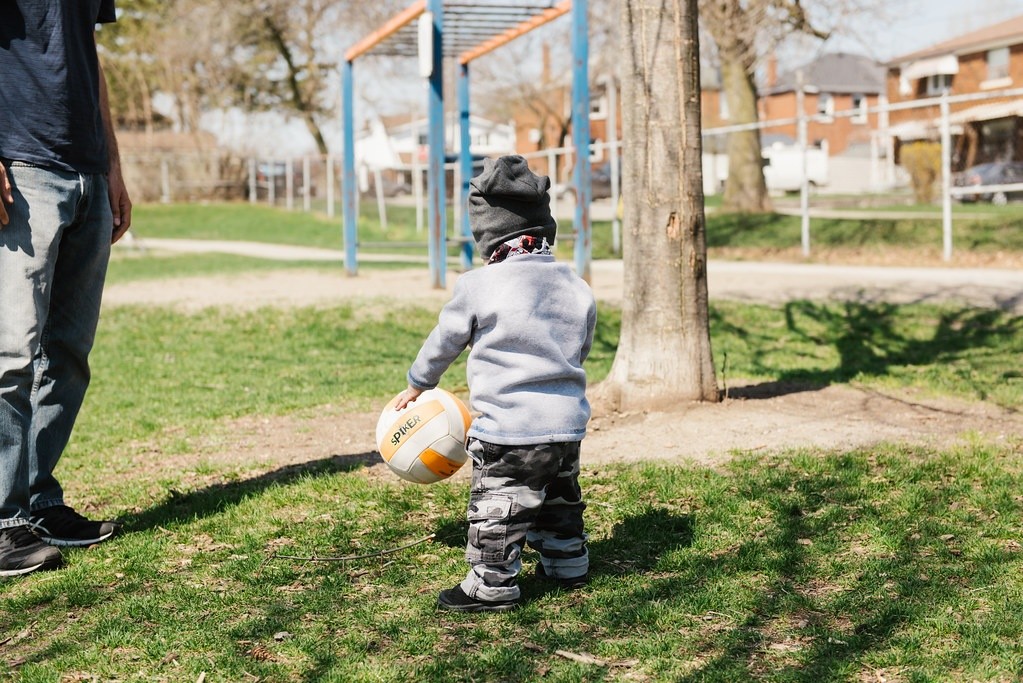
[390,153,600,613]
[0,0,132,578]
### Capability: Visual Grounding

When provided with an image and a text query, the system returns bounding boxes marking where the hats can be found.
[468,154,557,260]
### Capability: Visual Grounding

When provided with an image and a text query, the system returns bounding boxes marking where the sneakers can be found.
[0,526,62,576]
[30,504,120,546]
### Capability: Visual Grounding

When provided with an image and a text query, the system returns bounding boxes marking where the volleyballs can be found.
[375,387,473,484]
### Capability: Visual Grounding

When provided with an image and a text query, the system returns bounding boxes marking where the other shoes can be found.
[439,585,517,612]
[535,559,588,589]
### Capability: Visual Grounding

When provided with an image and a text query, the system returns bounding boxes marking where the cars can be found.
[368,174,412,198]
[548,166,612,204]
[241,161,303,201]
[760,132,830,197]
[952,160,1023,206]
